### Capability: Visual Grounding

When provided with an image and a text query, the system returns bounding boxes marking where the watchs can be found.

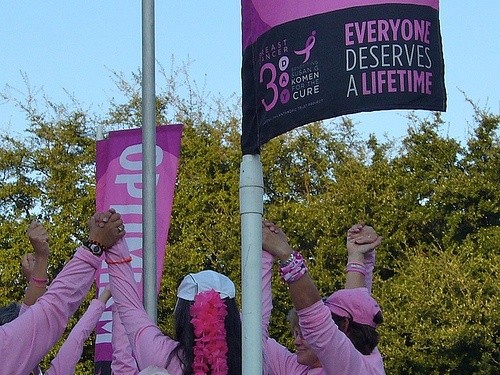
[277,250,295,268]
[83,242,104,256]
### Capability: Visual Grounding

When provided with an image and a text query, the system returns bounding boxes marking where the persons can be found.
[263,222,378,375]
[262,218,384,375]
[94,207,276,375]
[0,207,138,375]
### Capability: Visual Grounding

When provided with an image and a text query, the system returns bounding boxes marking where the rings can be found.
[367,235,372,239]
[117,226,124,232]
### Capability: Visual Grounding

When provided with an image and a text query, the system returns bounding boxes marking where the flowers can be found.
[189,288,228,375]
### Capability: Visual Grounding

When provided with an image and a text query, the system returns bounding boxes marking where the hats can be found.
[177,271,234,300]
[324,288,381,328]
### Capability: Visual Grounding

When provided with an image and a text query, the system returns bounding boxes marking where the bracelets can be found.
[27,274,49,284]
[88,239,108,252]
